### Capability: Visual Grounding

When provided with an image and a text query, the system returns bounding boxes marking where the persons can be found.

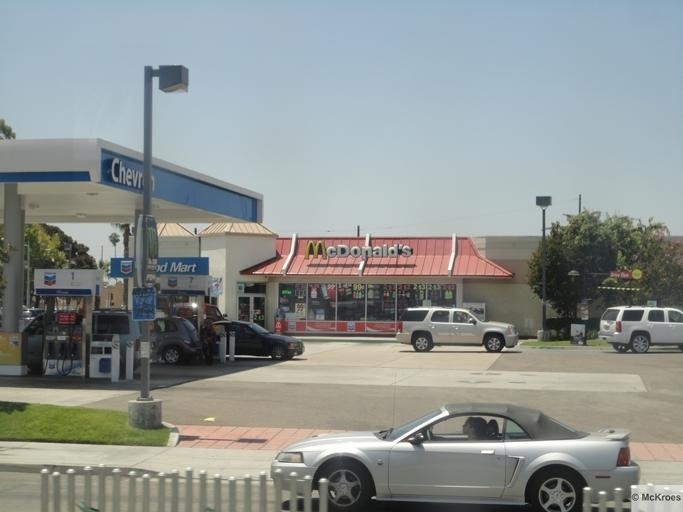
[461,417,479,440]
[547,324,585,345]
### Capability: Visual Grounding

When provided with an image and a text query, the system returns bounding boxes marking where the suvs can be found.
[395,305,520,352]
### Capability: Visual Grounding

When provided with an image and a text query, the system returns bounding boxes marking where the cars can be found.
[201,319,303,365]
[270,403,641,512]
[175,303,228,333]
[151,316,202,364]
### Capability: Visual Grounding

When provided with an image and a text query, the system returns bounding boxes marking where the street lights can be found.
[140,65,188,401]
[535,197,552,330]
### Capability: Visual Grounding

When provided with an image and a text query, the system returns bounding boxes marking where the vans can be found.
[21,309,141,376]
[597,304,683,353]
